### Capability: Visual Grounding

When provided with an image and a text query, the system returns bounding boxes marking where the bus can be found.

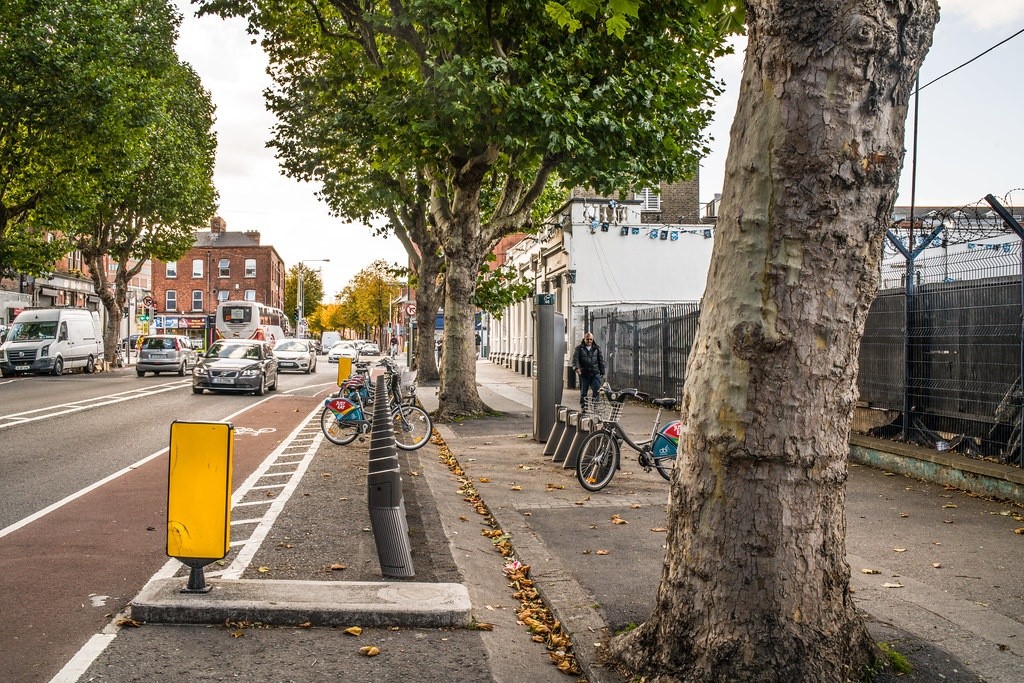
[213,300,291,342]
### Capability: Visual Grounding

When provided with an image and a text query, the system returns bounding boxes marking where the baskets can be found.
[584,396,625,423]
[400,379,418,399]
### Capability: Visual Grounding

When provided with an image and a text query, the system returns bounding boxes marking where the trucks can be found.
[322,331,341,355]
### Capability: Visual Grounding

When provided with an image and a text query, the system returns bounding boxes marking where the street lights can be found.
[296,258,331,321]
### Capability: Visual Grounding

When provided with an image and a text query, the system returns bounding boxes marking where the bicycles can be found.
[321,357,434,450]
[576,381,682,493]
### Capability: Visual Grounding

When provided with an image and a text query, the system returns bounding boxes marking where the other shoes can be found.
[395,352,397,354]
[391,357,393,358]
[476,355,478,360]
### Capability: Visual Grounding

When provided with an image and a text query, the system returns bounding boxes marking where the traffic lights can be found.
[140,316,150,321]
[293,308,299,321]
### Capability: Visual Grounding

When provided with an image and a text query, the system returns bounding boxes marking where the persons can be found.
[475,332,481,360]
[437,336,442,367]
[571,332,605,415]
[390,335,398,358]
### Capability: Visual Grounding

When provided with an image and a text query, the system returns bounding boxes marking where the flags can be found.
[588,199,711,241]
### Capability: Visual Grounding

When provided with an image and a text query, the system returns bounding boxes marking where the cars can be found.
[122,334,141,349]
[136,334,198,377]
[272,341,317,375]
[328,338,373,363]
[305,338,320,355]
[192,339,278,395]
[361,344,381,356]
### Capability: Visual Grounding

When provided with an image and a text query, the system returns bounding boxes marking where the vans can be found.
[0,309,98,377]
